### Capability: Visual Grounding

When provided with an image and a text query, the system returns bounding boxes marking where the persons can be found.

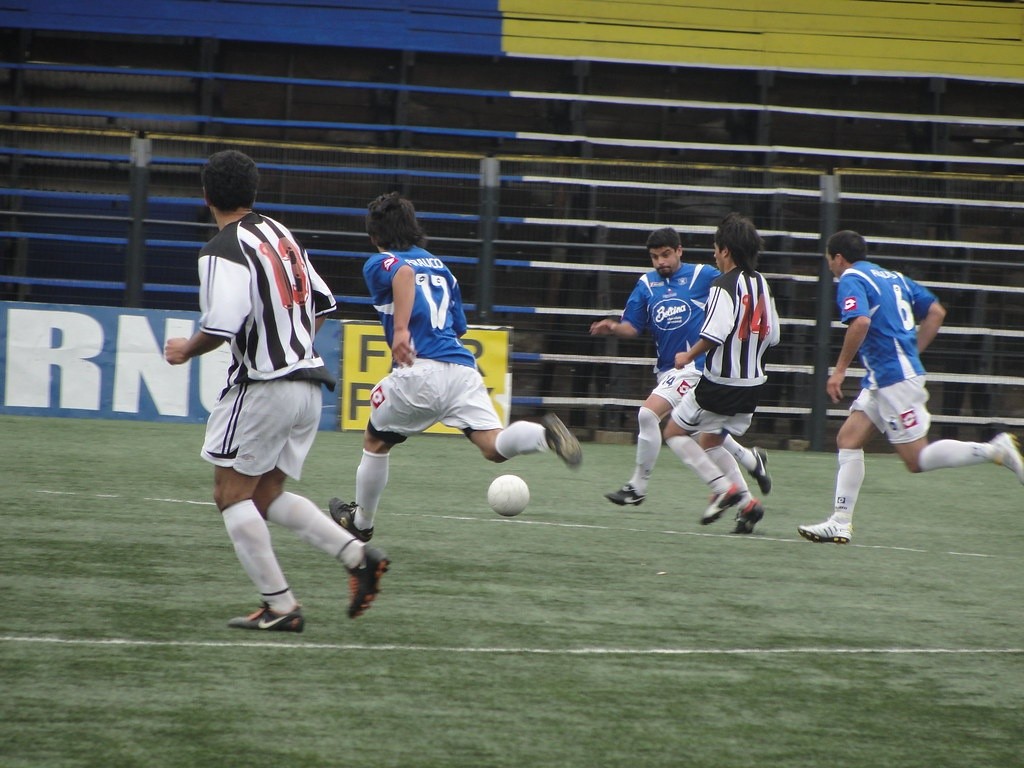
[588,227,774,505]
[163,149,392,634]
[329,190,585,544]
[660,210,781,535]
[796,229,1023,546]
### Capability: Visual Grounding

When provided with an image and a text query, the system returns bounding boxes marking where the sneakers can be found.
[748,446,771,496]
[328,497,374,541]
[796,519,854,545]
[540,411,583,470]
[604,483,648,506]
[227,598,304,634]
[730,498,764,534]
[700,482,743,525]
[989,431,1024,485]
[344,542,392,618]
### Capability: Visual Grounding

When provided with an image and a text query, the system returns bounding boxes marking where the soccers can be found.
[489,474,530,517]
